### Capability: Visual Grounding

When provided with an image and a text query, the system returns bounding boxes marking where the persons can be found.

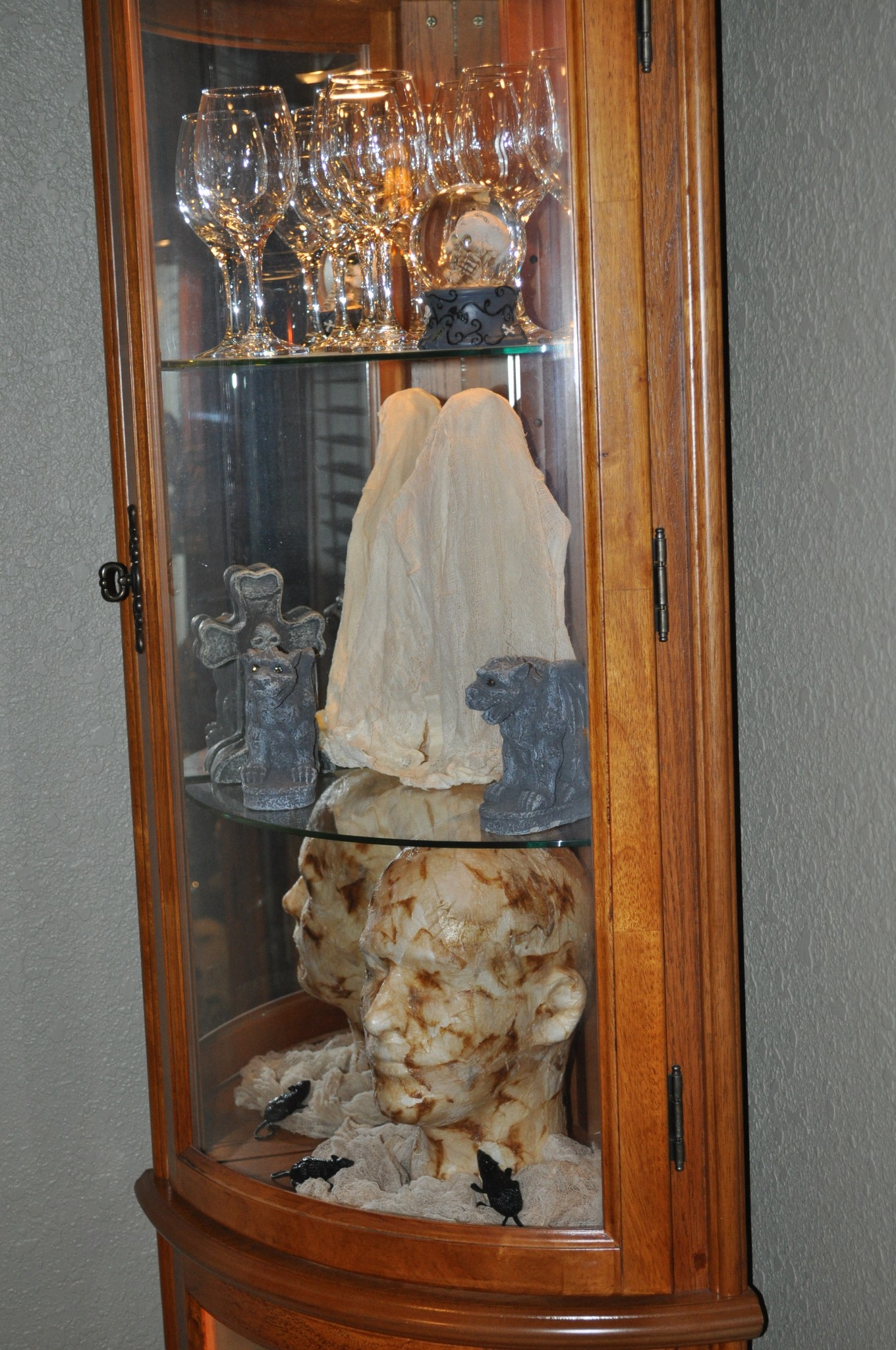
[292,843,604,1226]
[234,769,489,1142]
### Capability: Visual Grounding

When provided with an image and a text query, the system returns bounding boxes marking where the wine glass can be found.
[175,46,574,360]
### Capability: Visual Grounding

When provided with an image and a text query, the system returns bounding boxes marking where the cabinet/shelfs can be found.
[82,0,764,1350]
